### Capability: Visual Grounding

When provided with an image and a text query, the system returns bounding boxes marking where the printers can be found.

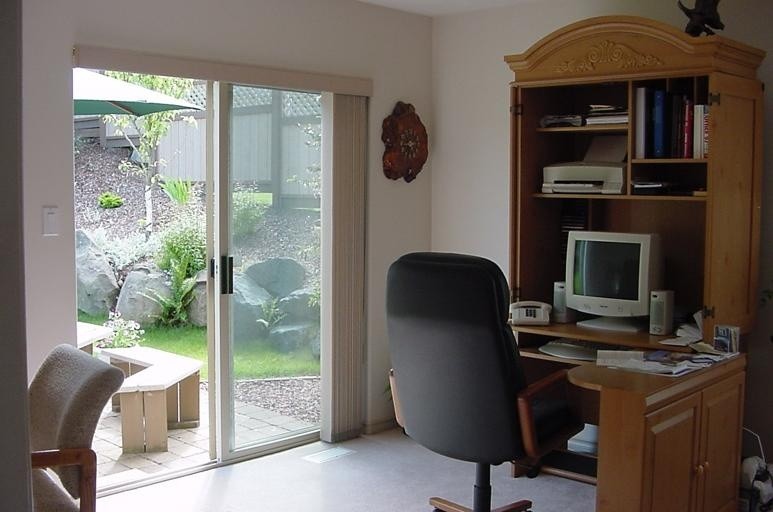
[542,159,628,196]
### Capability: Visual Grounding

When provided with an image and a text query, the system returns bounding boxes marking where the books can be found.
[595,341,717,380]
[538,104,630,128]
[633,83,709,162]
[712,324,742,352]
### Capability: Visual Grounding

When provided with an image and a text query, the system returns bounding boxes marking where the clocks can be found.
[382,101,428,183]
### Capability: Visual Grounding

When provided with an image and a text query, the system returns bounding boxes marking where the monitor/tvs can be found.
[566,231,665,333]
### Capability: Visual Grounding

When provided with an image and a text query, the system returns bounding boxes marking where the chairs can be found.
[28,341,126,512]
[388,251,586,512]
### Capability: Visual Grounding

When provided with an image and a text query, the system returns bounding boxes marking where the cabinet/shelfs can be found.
[499,16,769,512]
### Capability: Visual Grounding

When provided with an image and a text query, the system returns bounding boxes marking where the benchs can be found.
[76,322,205,454]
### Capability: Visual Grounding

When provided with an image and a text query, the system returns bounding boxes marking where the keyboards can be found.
[539,338,625,361]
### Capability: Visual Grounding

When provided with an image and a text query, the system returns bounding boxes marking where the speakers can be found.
[554,282,573,324]
[649,290,674,336]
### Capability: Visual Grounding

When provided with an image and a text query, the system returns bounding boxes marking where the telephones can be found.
[509,301,552,325]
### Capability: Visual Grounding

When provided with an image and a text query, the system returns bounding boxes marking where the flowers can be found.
[97,306,146,348]
